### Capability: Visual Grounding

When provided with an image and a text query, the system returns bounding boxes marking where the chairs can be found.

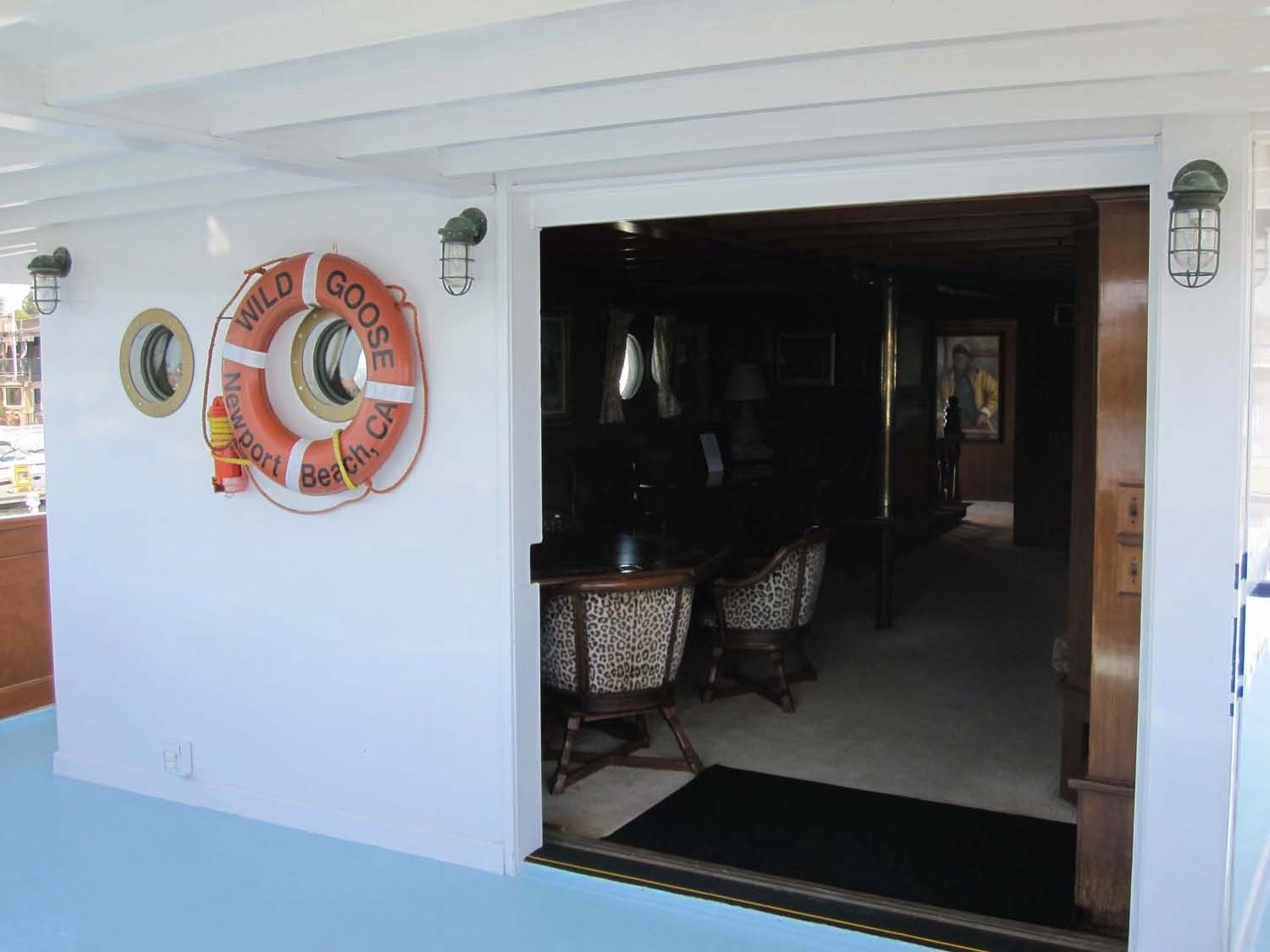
[541,569,699,793]
[704,517,833,713]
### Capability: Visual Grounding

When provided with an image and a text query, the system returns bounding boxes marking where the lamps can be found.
[27,246,72,315]
[720,361,773,458]
[439,207,489,297]
[1168,160,1227,288]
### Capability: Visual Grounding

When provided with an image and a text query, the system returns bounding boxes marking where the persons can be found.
[936,342,999,431]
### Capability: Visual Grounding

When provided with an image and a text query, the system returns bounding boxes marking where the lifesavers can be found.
[223,250,415,496]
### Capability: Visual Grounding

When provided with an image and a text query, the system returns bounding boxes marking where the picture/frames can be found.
[776,331,837,384]
[541,309,573,427]
[935,330,1005,440]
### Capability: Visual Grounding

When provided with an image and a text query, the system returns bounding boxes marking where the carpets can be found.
[601,764,1078,932]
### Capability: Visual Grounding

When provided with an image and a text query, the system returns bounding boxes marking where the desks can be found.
[543,530,729,585]
